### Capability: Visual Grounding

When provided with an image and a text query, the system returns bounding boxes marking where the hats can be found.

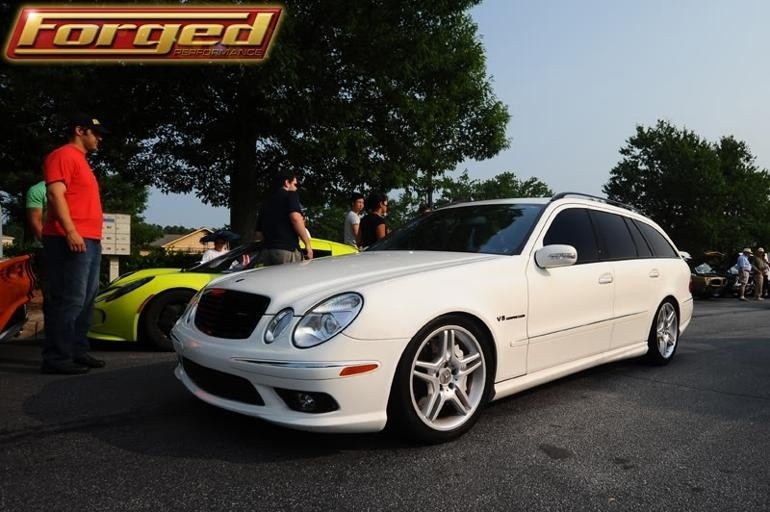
[743,247,753,254]
[68,114,105,134]
[757,247,764,252]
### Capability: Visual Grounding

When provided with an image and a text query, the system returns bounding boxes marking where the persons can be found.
[23,180,51,300]
[254,168,316,267]
[343,193,366,250]
[751,247,767,301]
[36,109,109,376]
[198,234,240,271]
[736,247,754,302]
[355,190,389,248]
[761,258,770,300]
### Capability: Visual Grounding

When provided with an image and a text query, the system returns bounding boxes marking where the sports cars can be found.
[0,254,37,341]
[87,238,359,351]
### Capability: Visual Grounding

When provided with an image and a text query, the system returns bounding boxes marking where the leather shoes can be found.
[40,360,89,374]
[76,353,106,368]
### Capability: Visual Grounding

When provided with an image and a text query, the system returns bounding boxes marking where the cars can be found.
[681,252,754,298]
[171,192,695,442]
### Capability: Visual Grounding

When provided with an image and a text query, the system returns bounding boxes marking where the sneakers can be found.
[754,298,765,301]
[739,297,749,301]
